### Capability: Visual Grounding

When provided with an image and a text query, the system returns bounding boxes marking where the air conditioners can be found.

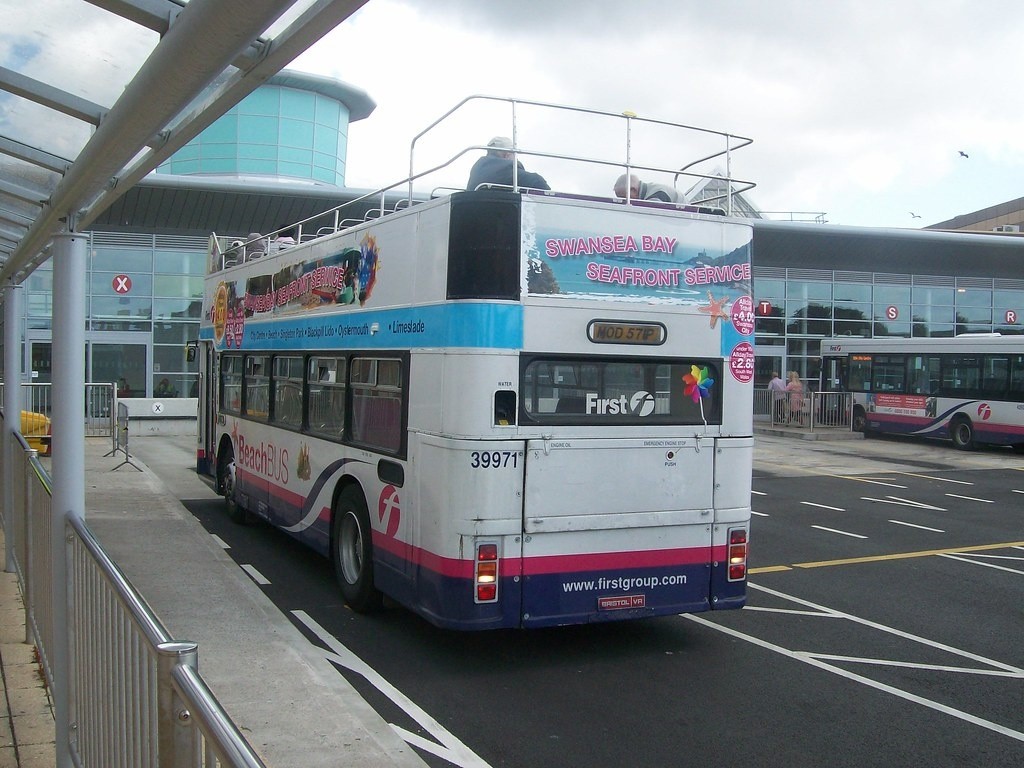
[1001,225,1019,233]
[992,227,1002,231]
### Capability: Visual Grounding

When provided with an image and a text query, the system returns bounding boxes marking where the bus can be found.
[194,95,757,633]
[816,333,1024,455]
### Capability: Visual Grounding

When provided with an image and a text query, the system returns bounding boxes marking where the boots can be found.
[796,416,805,429]
[785,416,793,427]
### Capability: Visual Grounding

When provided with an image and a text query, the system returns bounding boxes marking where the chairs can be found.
[225,186,727,272]
[553,396,594,412]
[248,388,404,452]
[876,375,1024,392]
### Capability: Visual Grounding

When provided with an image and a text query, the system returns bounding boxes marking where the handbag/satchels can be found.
[801,401,805,407]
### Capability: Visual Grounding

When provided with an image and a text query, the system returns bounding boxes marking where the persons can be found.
[331,260,360,305]
[113,379,131,398]
[615,174,690,206]
[467,138,551,193]
[237,232,267,266]
[784,371,805,428]
[155,379,177,398]
[261,225,296,257]
[217,241,244,271]
[767,372,786,425]
[867,395,876,413]
